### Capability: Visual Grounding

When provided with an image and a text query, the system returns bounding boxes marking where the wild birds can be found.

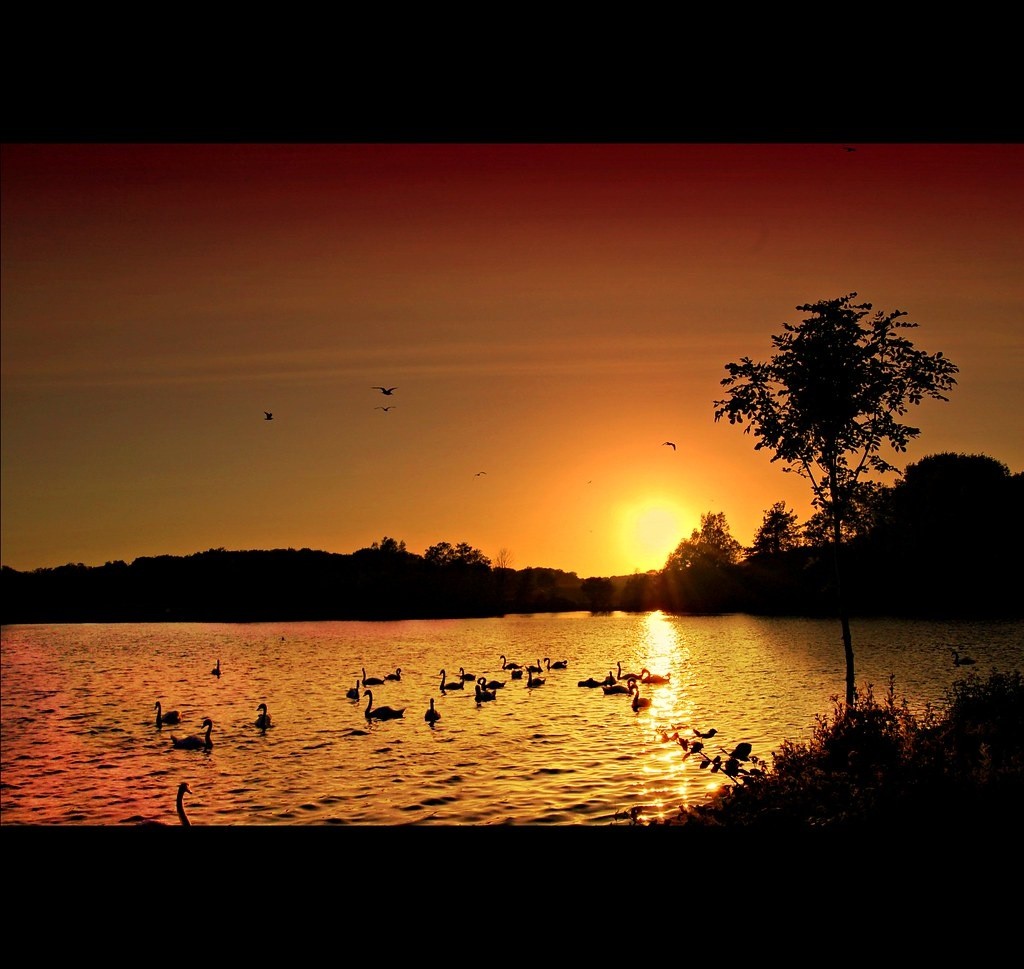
[370,386,398,395]
[263,412,273,421]
[951,651,974,664]
[842,147,856,153]
[255,703,272,728]
[153,702,181,724]
[170,719,213,749]
[374,406,396,411]
[347,655,568,722]
[211,660,221,675]
[136,781,192,826]
[577,662,670,708]
[474,472,486,477]
[662,442,676,450]
